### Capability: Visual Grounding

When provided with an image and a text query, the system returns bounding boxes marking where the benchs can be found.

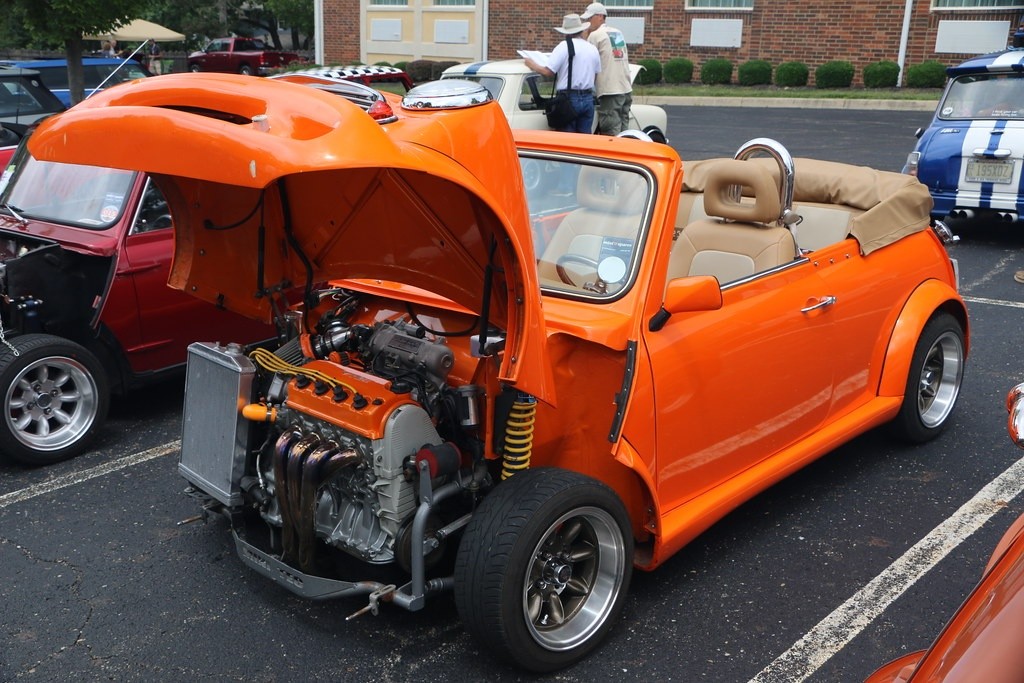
[671,158,934,257]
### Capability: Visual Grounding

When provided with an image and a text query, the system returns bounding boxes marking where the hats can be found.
[553,14,590,34]
[580,3,607,19]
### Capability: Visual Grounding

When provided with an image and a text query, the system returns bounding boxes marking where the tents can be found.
[82,13,187,57]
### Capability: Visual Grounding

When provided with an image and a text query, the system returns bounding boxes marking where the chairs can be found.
[536,164,648,293]
[661,159,794,285]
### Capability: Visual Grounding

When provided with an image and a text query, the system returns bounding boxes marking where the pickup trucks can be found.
[187,37,300,77]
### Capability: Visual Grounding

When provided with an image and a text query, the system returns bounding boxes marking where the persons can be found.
[580,2,633,137]
[147,38,163,75]
[103,40,118,58]
[523,14,602,134]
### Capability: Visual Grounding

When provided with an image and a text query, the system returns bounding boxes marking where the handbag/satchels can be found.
[543,94,577,130]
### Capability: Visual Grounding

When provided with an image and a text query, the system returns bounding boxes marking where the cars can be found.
[277,65,415,95]
[0,113,332,466]
[900,25,1024,226]
[859,381,1024,682]
[0,65,69,180]
[440,59,669,200]
[0,58,154,109]
[24,73,972,680]
